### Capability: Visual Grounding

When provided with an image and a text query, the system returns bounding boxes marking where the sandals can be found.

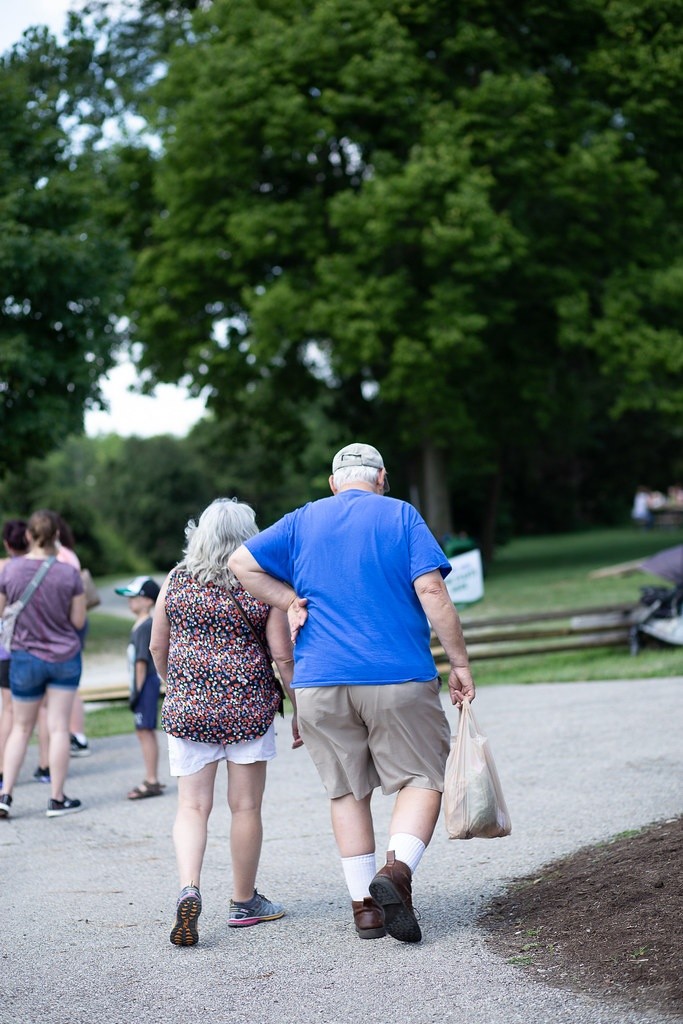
[129,780,165,800]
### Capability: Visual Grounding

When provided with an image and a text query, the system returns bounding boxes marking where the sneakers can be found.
[34,767,52,782]
[168,885,201,947]
[69,735,90,757]
[0,794,11,816]
[228,888,285,927]
[46,792,87,817]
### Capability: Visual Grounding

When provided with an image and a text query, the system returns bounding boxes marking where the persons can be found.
[0,516,49,783]
[148,493,305,948]
[44,515,102,756]
[228,442,475,942]
[116,573,166,798]
[631,483,683,529]
[1,506,87,816]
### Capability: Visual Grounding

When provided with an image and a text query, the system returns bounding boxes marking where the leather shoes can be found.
[369,851,423,943]
[350,901,385,940]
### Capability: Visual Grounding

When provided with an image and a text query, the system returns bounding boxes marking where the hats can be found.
[332,441,391,493]
[115,576,160,603]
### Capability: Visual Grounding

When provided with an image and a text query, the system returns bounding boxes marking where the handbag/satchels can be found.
[442,693,512,839]
[3,600,25,653]
